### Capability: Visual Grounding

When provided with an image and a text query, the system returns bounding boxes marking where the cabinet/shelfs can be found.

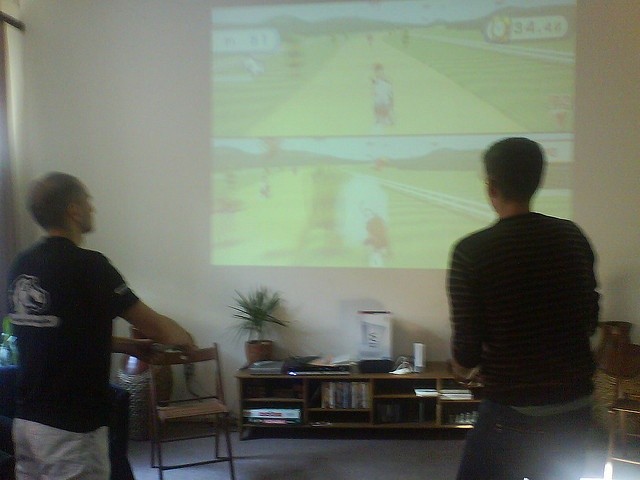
[238,360,485,440]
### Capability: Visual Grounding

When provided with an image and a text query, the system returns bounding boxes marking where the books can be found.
[318,379,371,409]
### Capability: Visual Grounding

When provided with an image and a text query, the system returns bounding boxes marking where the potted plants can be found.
[228,284,291,368]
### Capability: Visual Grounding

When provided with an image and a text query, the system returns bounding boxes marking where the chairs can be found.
[145,343,237,480]
[602,348,639,468]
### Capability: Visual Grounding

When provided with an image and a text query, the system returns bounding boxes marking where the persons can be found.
[445,136,601,476]
[6,172,195,480]
